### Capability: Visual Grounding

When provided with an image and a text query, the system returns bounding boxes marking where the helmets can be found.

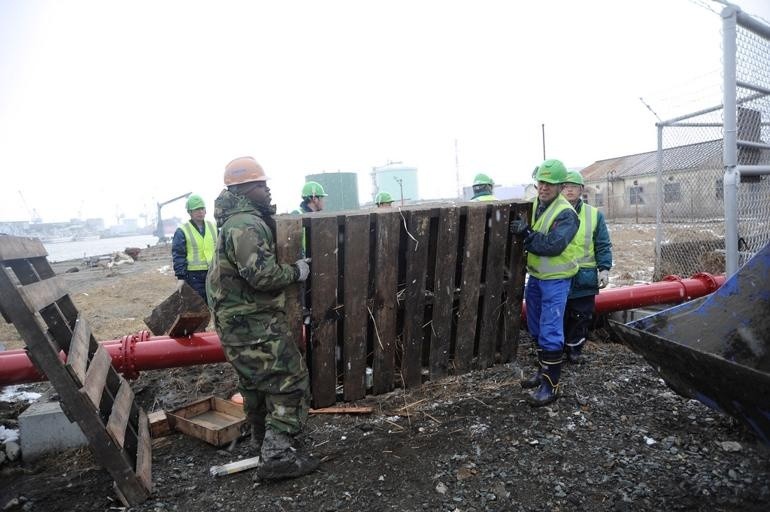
[301,181,328,197]
[535,159,585,186]
[375,192,395,205]
[224,156,267,186]
[185,195,206,211]
[472,174,495,186]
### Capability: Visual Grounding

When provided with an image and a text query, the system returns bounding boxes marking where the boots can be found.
[521,338,586,407]
[249,423,319,481]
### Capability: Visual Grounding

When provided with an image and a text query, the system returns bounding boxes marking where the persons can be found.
[291,182,327,307]
[205,159,321,484]
[508,158,580,407]
[469,173,501,203]
[374,193,396,209]
[561,170,612,364]
[172,196,221,309]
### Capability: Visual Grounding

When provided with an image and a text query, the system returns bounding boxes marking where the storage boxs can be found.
[167,395,252,447]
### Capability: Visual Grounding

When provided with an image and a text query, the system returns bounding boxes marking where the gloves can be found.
[178,279,184,293]
[509,213,529,234]
[295,258,311,281]
[598,270,608,288]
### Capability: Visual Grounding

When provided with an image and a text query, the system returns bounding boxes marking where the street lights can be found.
[394,177,404,207]
[606,170,612,224]
[610,169,617,224]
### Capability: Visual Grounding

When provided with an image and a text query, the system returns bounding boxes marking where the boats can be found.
[39,232,101,244]
